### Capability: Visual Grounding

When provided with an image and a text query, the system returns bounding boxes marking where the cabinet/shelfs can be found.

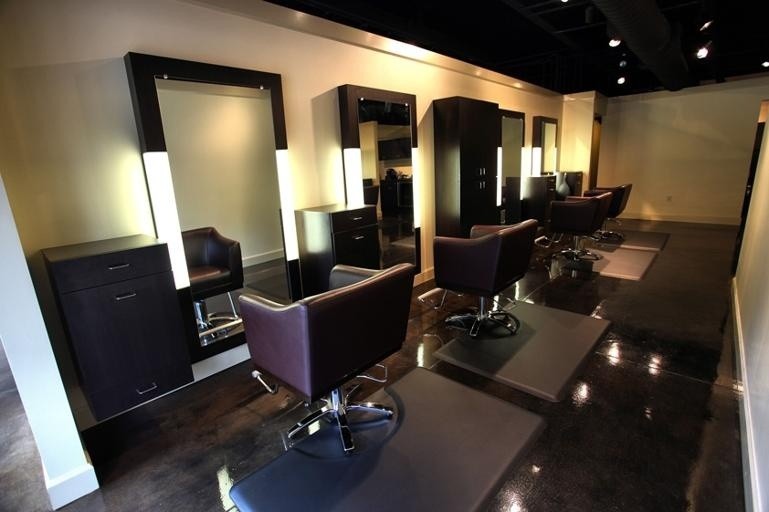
[41,233,195,422]
[294,203,379,298]
[432,96,499,239]
[527,176,556,233]
[561,171,583,196]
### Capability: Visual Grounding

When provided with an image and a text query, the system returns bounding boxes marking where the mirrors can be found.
[124,51,302,365]
[496,108,525,211]
[339,83,422,275]
[531,116,558,177]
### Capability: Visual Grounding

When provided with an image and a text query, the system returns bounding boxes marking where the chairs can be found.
[417,217,539,337]
[534,191,614,262]
[237,261,416,453]
[584,184,632,243]
[181,227,244,340]
